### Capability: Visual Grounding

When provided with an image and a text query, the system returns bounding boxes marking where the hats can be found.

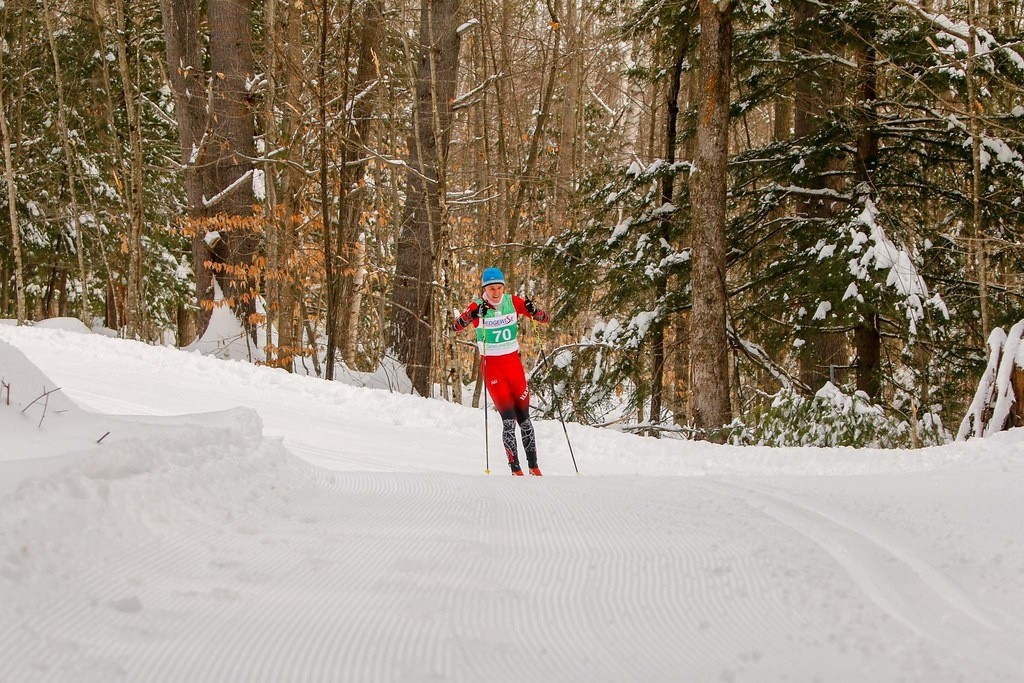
[481,268,505,288]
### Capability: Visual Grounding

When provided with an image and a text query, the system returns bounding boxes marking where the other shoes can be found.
[529,467,542,477]
[512,469,524,476]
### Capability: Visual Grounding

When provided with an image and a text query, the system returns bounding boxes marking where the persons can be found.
[451,267,548,476]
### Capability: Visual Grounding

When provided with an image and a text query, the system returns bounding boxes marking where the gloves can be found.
[471,302,490,320]
[523,295,536,315]
